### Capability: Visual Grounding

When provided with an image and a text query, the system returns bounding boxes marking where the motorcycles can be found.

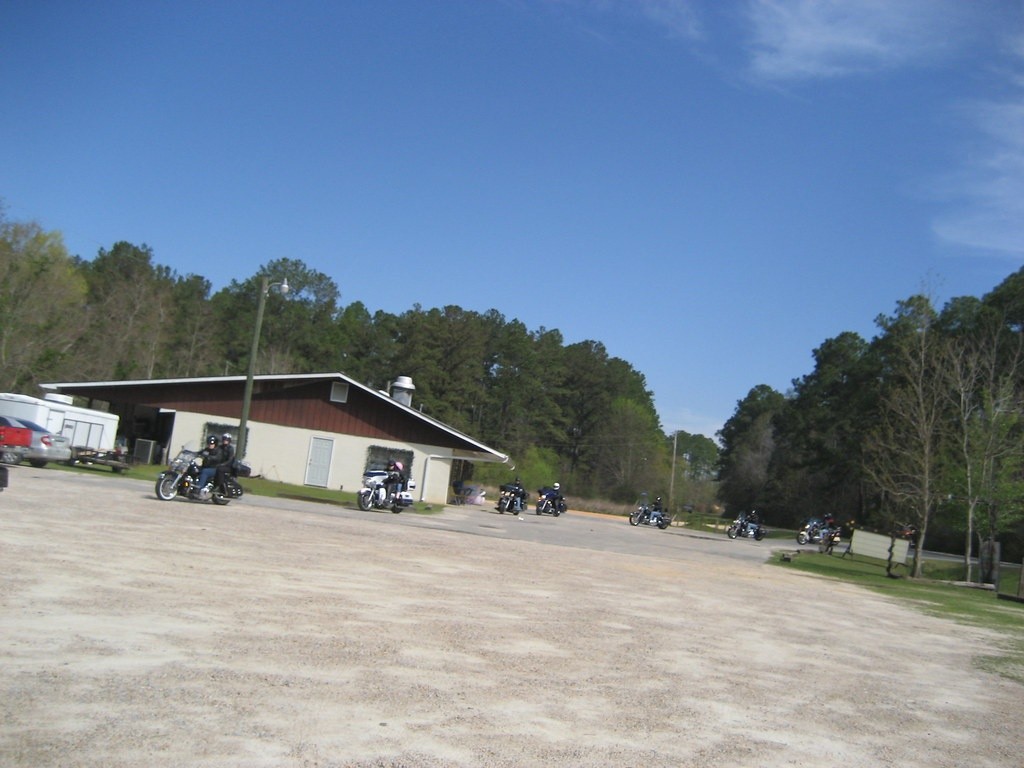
[355,462,416,514]
[154,440,252,506]
[797,519,842,545]
[629,504,672,529]
[535,488,568,517]
[495,484,531,516]
[727,514,766,541]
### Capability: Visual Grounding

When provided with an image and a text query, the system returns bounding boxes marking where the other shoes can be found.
[210,485,220,492]
[383,498,390,503]
[392,498,400,503]
[194,486,201,494]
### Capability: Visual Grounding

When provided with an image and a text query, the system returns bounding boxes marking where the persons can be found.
[540,482,564,512]
[384,460,409,503]
[745,510,759,534]
[647,496,663,522]
[816,512,842,554]
[501,476,526,509]
[191,432,236,493]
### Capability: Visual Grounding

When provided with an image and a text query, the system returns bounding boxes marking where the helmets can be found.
[553,483,560,490]
[387,460,397,471]
[515,477,521,485]
[221,433,232,444]
[751,510,757,517]
[827,513,832,518]
[207,435,218,446]
[656,497,661,502]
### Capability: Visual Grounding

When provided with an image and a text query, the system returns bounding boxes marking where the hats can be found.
[396,462,404,470]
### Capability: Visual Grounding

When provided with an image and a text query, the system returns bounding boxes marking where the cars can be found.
[0,415,73,468]
